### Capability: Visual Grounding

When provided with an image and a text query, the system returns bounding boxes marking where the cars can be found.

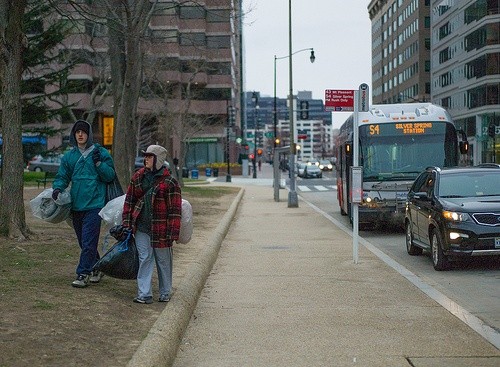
[27,152,65,173]
[297,157,336,178]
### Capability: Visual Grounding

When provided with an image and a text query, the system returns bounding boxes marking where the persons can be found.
[122,146,182,303]
[52,120,114,287]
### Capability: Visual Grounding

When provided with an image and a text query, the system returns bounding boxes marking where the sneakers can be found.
[158,294,171,301]
[72,273,90,287]
[133,296,153,303]
[90,270,104,282]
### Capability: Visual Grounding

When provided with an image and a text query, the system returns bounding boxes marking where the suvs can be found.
[405,163,500,271]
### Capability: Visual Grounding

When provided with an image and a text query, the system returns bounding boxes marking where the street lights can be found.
[252,92,259,178]
[274,48,316,147]
[223,92,236,182]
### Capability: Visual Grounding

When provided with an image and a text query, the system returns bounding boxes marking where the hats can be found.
[74,122,89,137]
[141,145,167,170]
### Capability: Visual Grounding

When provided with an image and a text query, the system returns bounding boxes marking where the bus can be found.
[337,103,469,231]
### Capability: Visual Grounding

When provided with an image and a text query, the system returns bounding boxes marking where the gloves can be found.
[92,149,101,164]
[52,189,62,200]
